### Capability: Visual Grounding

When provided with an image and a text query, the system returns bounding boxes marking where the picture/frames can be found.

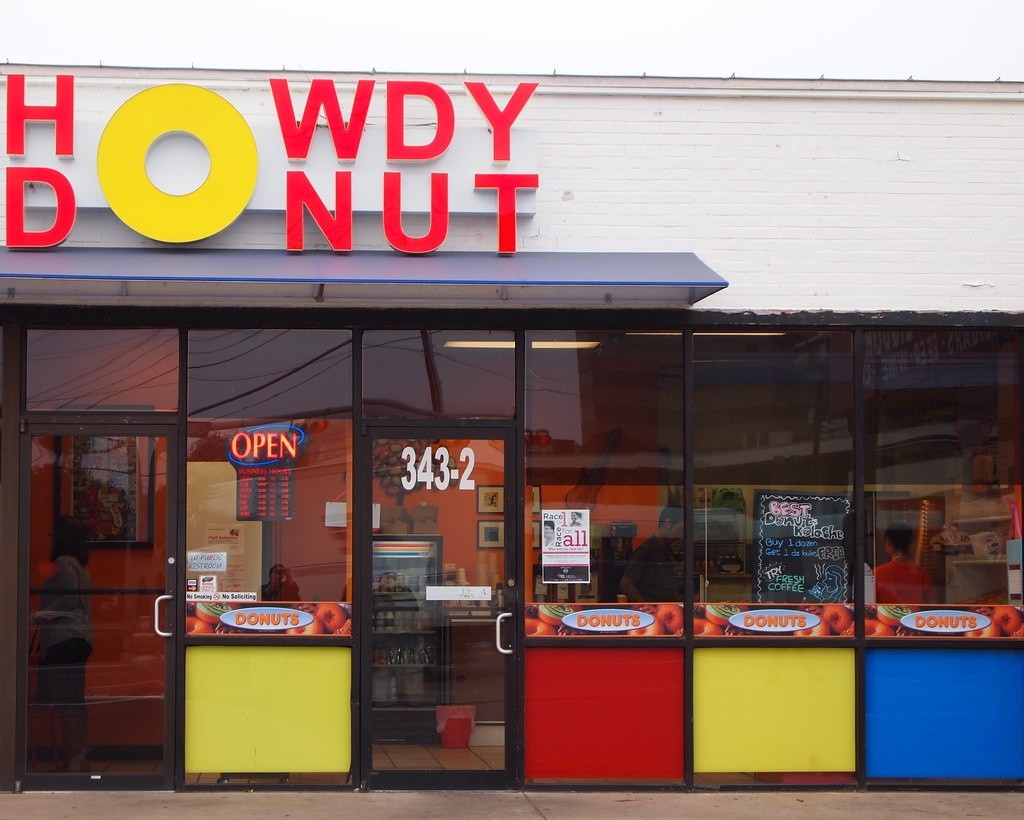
[55,402,156,551]
[477,485,542,513]
[477,520,541,549]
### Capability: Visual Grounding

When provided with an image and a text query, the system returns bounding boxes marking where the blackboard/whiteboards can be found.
[752,488,854,603]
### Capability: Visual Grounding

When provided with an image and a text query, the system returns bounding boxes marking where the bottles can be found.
[394,611,403,631]
[403,611,413,630]
[412,611,423,629]
[532,554,550,602]
[494,583,504,618]
[387,676,398,702]
[385,611,394,631]
[376,610,385,631]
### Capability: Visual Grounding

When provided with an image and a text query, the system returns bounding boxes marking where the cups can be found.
[477,551,498,590]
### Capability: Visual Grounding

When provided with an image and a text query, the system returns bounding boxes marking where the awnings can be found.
[0,251,730,311]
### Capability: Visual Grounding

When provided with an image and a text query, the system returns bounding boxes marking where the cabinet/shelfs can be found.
[694,506,746,578]
[943,484,1013,607]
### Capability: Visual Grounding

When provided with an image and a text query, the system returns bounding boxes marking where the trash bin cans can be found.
[436,706,476,749]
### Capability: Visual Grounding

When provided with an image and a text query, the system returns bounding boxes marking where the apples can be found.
[865,620,896,636]
[626,604,685,636]
[525,617,556,637]
[963,607,1023,638]
[792,606,853,638]
[693,618,724,636]
[186,615,215,634]
[288,604,348,635]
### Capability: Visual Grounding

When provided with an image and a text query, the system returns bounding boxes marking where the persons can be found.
[872,522,940,611]
[371,571,426,705]
[569,512,582,526]
[545,520,554,547]
[618,504,685,601]
[242,564,301,609]
[29,514,92,775]
